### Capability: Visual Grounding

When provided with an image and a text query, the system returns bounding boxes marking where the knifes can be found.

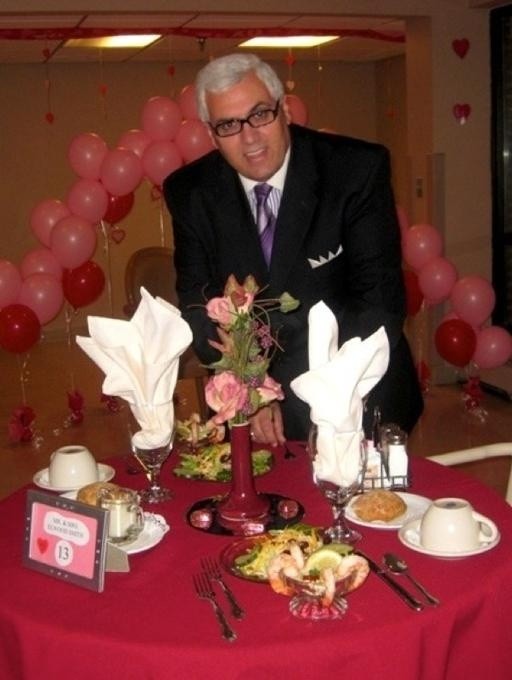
[354,549,424,612]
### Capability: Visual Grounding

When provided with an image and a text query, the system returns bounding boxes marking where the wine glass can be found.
[305,425,367,547]
[127,424,175,505]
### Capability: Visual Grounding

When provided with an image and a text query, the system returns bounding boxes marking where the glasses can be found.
[208,98,280,138]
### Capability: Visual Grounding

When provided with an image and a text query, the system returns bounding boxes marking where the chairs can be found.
[121,246,208,422]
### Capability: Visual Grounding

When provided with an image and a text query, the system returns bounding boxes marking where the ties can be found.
[255,183,277,270]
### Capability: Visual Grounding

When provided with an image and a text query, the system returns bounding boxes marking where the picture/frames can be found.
[16,487,131,596]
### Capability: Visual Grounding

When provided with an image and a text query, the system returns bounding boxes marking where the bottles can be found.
[376,420,410,486]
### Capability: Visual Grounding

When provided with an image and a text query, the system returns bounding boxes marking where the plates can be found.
[32,463,115,493]
[221,533,278,584]
[107,513,163,554]
[56,489,80,502]
[342,490,433,529]
[398,513,502,561]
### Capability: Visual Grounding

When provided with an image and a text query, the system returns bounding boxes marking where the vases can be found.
[215,420,271,533]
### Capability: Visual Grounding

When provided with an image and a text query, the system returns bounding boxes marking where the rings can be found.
[250,432,254,436]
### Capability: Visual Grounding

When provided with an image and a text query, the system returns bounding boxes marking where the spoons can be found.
[380,550,441,606]
[282,444,297,461]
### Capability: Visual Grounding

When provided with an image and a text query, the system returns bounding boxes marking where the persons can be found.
[162,52,424,452]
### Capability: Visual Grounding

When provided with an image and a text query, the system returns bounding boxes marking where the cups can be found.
[49,445,99,487]
[98,490,144,546]
[419,498,497,553]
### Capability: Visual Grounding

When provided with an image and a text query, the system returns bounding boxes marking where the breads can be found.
[350,488,409,523]
[76,481,119,505]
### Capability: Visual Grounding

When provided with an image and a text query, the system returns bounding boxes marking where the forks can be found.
[198,556,246,620]
[191,571,239,641]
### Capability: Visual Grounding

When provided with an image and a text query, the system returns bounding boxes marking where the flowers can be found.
[198,273,301,424]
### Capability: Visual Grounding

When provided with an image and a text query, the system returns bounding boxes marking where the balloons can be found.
[2,73,215,355]
[282,93,307,127]
[395,203,511,386]
